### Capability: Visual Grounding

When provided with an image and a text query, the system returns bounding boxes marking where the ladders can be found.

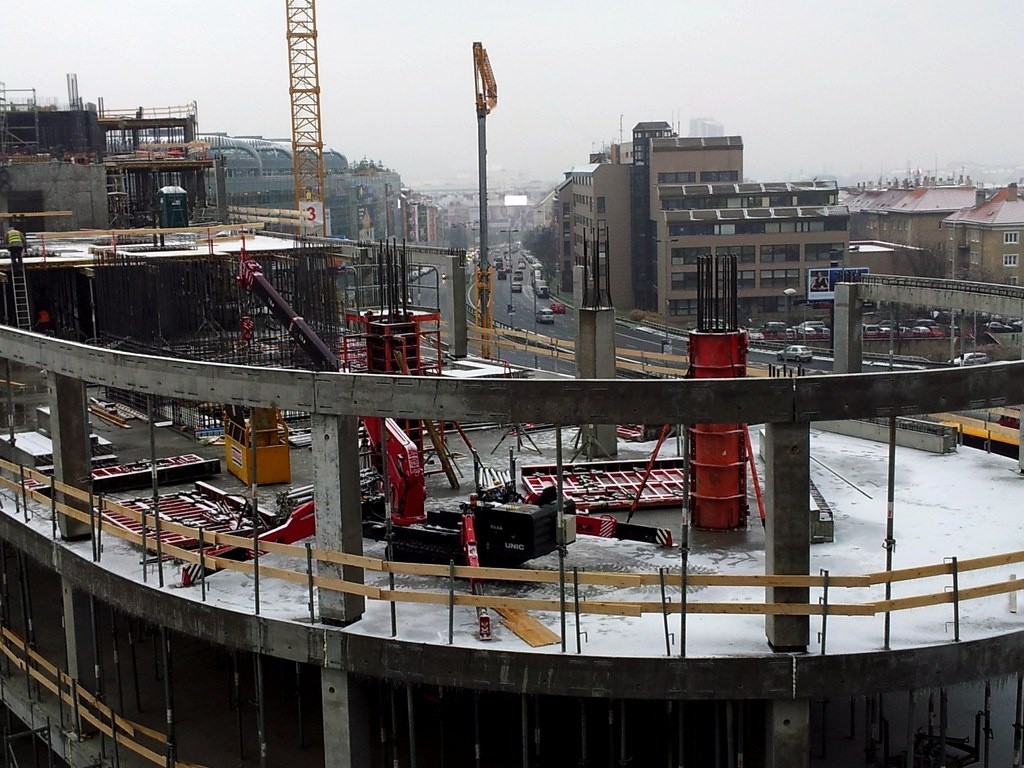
[10,263,32,331]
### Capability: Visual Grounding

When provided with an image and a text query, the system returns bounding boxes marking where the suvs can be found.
[536,309,554,324]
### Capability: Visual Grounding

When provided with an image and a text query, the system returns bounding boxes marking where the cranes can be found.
[286,0,326,237]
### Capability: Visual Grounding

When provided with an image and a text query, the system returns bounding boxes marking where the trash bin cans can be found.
[661,339,673,354]
[157,186,189,228]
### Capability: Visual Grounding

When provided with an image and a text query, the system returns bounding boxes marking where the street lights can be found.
[500,229,519,330]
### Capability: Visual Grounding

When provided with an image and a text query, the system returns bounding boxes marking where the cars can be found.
[776,345,813,362]
[550,302,565,313]
[694,315,1024,338]
[466,241,552,295]
[948,353,989,366]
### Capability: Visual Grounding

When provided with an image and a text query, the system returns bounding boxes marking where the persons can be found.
[810,271,828,291]
[4,226,27,274]
